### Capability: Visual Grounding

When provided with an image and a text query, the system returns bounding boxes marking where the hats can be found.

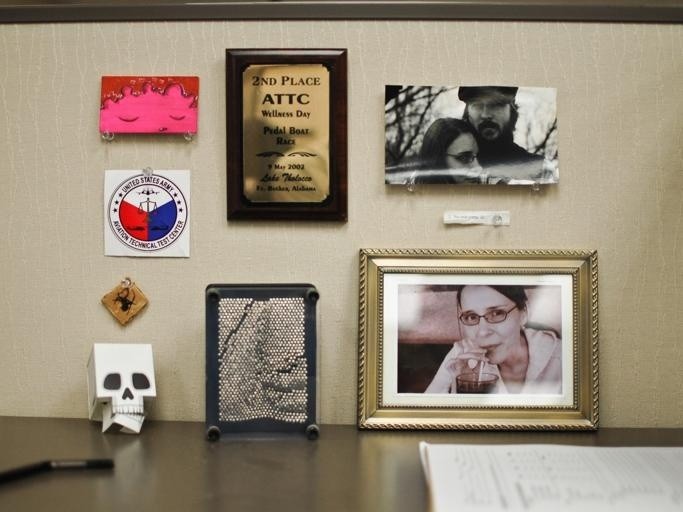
[457,86,518,103]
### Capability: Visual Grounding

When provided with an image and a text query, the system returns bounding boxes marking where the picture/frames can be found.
[356,248,601,432]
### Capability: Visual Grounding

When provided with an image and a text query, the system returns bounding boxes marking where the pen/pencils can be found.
[0,459,114,484]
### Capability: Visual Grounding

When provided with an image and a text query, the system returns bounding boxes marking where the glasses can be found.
[446,151,485,165]
[458,304,518,327]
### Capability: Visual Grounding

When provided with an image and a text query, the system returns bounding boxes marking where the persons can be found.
[422,284,561,398]
[409,117,485,187]
[455,88,550,184]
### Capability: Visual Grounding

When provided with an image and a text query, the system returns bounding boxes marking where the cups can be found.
[454,370,500,394]
